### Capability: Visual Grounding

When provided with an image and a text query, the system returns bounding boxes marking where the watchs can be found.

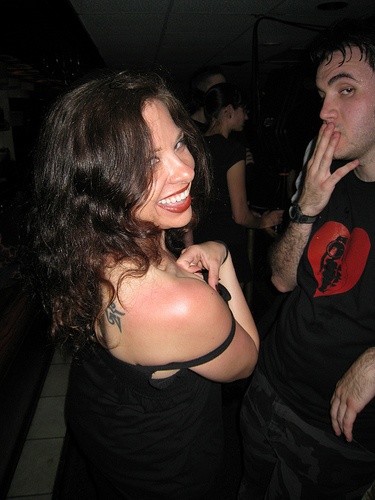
[288,202,328,223]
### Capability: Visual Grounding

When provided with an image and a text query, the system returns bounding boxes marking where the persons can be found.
[16,70,260,499]
[189,68,284,285]
[239,36,374,499]
